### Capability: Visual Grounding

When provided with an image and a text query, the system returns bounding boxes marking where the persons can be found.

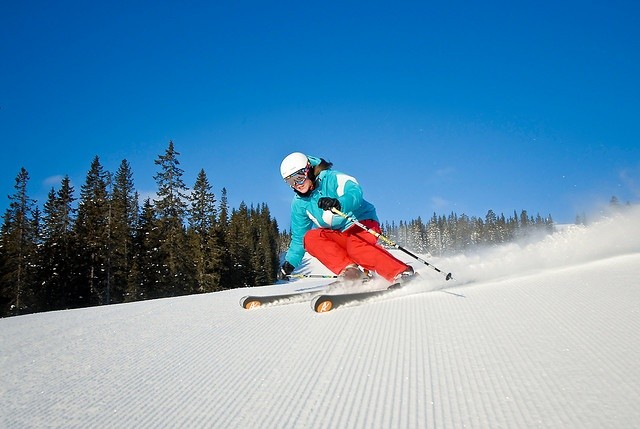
[279,151,421,291]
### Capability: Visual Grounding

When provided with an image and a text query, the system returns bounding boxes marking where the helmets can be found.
[280,152,308,180]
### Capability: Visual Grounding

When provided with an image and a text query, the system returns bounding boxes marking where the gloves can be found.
[317,197,341,214]
[277,261,295,281]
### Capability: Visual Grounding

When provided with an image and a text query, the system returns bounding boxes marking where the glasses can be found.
[284,159,311,187]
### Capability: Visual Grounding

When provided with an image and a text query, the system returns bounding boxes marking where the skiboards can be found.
[240,278,396,313]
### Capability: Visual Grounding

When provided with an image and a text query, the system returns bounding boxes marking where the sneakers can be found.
[387,266,414,291]
[342,266,370,282]
